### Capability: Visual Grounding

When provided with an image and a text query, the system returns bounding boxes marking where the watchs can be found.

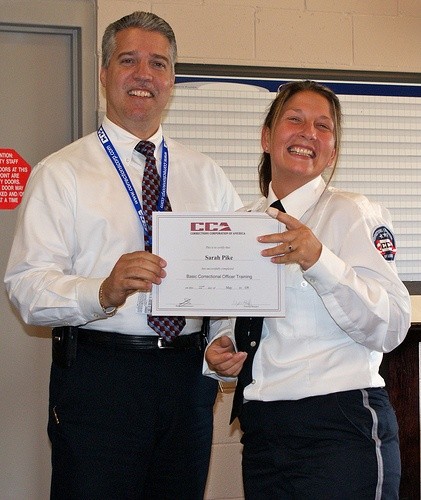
[99,282,117,318]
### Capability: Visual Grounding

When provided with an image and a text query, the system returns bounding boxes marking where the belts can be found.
[75,328,202,350]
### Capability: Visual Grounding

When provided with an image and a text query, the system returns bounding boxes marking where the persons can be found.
[2,11,248,500]
[199,77,412,500]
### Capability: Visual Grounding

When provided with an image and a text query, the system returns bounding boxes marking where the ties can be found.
[229,200,286,424]
[133,141,187,342]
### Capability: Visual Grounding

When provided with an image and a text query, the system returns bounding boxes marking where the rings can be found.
[287,241,292,253]
